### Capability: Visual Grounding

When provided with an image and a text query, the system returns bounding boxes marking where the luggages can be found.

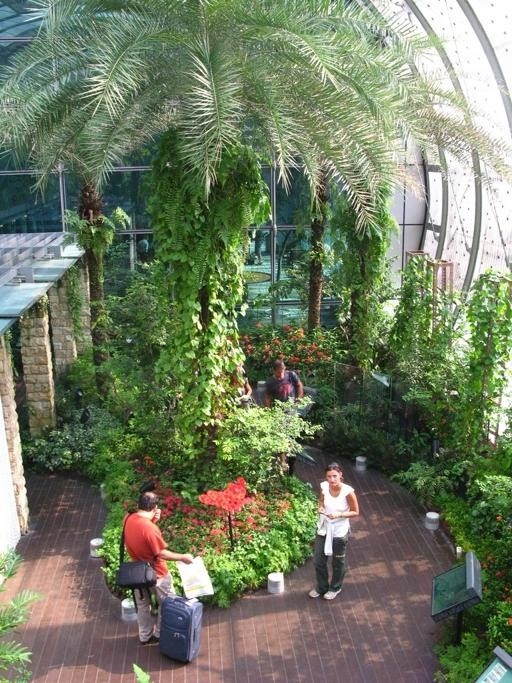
[160,595,203,664]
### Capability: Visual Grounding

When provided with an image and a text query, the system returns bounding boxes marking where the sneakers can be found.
[139,636,160,647]
[324,587,342,600]
[308,588,321,598]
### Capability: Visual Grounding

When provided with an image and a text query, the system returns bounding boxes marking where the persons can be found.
[250,218,270,265]
[118,487,195,645]
[308,461,359,601]
[263,359,306,477]
[233,365,252,409]
[136,234,150,260]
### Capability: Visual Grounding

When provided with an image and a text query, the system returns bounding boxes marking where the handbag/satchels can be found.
[115,561,157,590]
[175,556,214,599]
